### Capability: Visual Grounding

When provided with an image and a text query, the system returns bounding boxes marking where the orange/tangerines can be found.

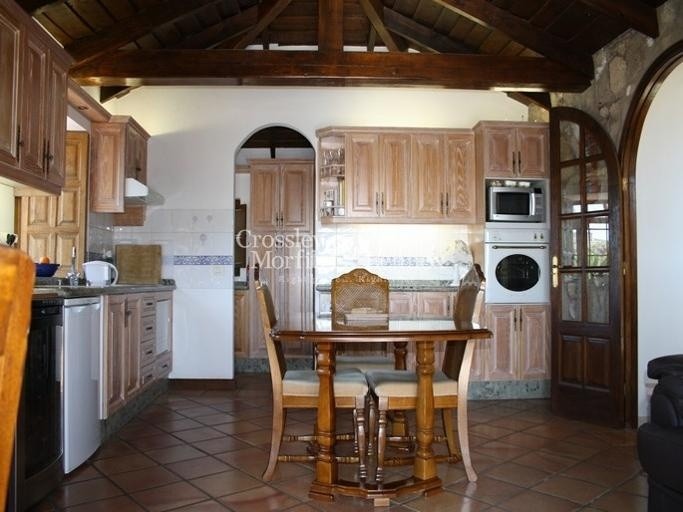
[40,256,50,264]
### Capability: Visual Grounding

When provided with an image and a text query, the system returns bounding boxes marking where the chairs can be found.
[375,264,486,490]
[253,264,369,488]
[328,268,391,360]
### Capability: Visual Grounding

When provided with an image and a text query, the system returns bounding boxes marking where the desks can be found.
[270,318,493,503]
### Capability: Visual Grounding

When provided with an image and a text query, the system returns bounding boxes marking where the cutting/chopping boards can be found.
[114,244,160,285]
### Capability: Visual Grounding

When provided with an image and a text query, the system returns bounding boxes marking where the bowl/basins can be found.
[32,262,60,277]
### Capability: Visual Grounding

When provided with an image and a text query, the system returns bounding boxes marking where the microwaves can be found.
[486,186,544,222]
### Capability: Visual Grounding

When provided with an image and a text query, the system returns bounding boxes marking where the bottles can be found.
[321,147,344,177]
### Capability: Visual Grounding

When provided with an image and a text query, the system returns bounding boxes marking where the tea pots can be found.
[80,260,119,287]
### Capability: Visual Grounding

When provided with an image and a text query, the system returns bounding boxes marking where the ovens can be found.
[14,311,65,503]
[484,223,550,304]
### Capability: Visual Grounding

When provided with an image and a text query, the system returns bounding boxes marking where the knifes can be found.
[4,232,17,246]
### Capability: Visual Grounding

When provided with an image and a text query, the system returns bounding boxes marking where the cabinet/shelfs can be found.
[486,303,553,381]
[347,128,474,225]
[417,290,484,379]
[0,0,71,196]
[141,292,156,395]
[320,292,414,364]
[104,293,141,419]
[475,121,550,177]
[247,158,316,359]
[90,115,150,227]
[315,125,347,225]
